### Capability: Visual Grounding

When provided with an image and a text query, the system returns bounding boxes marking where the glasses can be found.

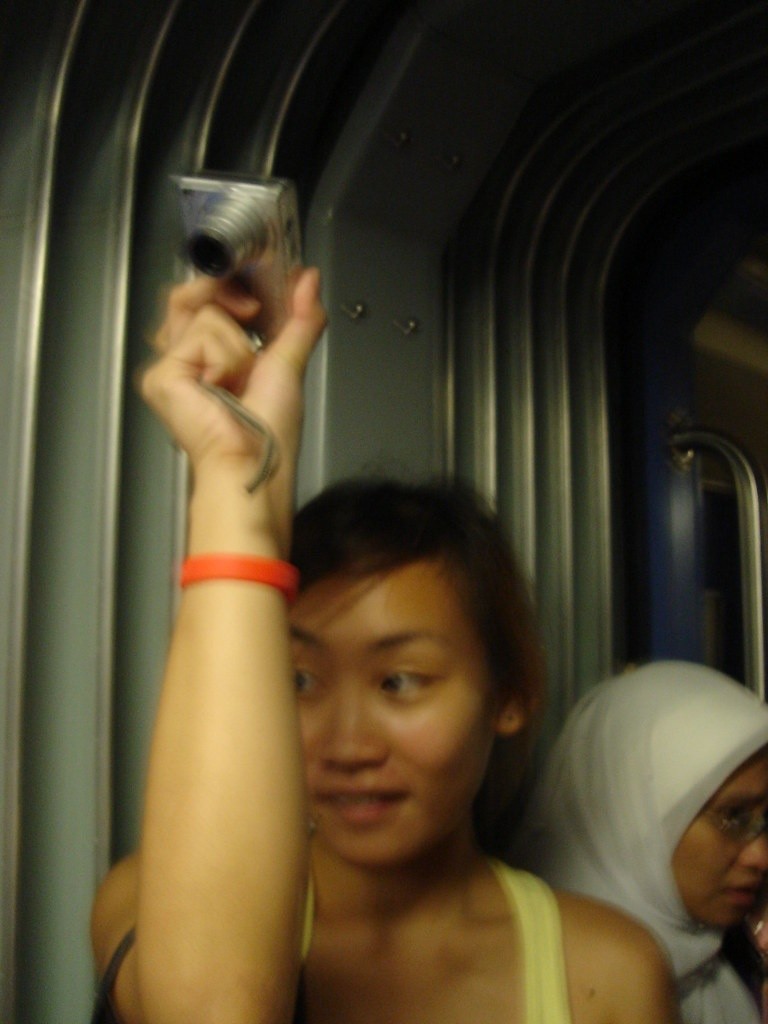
[700,806,768,842]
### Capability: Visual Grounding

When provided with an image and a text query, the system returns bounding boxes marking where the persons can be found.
[510,660,768,1024]
[90,263,681,1024]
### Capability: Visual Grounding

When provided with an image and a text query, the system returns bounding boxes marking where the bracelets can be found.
[179,555,301,605]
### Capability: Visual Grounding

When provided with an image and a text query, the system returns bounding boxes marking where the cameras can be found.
[175,171,307,346]
